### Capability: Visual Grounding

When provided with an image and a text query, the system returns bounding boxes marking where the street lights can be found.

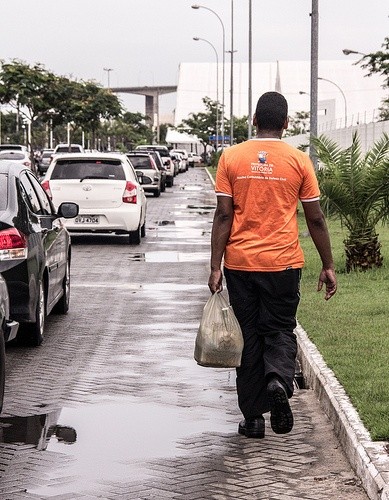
[316,77,347,131]
[191,3,224,153]
[191,36,219,152]
[104,66,114,88]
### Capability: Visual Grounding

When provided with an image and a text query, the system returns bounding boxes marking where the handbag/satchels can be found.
[194,290,244,367]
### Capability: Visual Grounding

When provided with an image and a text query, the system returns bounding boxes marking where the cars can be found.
[39,152,149,243]
[0,159,80,348]
[0,272,20,416]
[0,144,100,179]
[125,144,202,197]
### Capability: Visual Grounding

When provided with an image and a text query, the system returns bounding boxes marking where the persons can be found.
[34,146,42,174]
[208,91,337,439]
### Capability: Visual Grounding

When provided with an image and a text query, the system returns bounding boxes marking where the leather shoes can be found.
[238,416,265,438]
[266,378,294,434]
